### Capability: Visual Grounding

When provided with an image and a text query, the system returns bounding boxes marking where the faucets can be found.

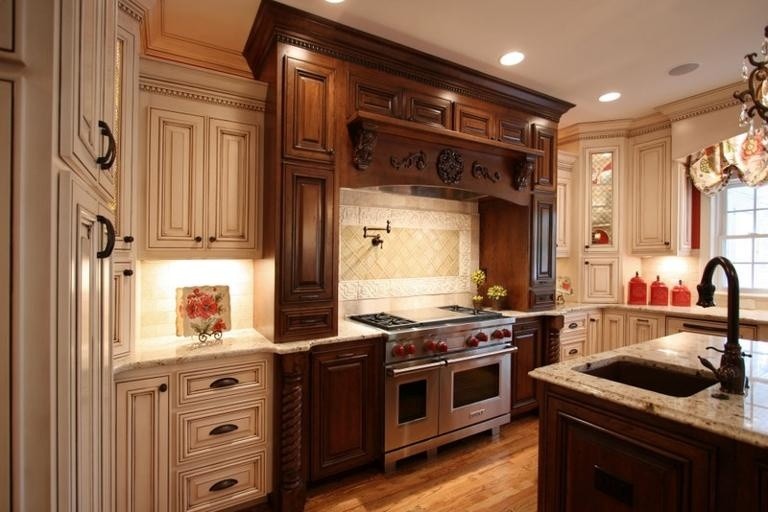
[695,255,753,396]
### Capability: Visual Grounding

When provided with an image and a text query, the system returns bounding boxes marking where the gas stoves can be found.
[348,304,502,329]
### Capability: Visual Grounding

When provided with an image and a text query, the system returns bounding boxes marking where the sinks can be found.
[572,355,722,397]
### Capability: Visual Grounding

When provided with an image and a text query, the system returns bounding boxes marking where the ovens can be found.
[384,339,519,452]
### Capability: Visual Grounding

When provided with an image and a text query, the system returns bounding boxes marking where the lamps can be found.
[733,24,768,139]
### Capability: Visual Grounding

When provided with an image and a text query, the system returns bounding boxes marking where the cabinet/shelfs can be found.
[560,311,603,361]
[626,123,702,257]
[252,44,338,344]
[603,308,665,352]
[557,165,572,259]
[113,353,275,512]
[305,336,381,485]
[478,123,558,313]
[346,61,453,133]
[453,90,532,147]
[535,380,718,511]
[135,54,268,259]
[511,317,543,421]
[13,1,118,512]
[574,141,623,304]
